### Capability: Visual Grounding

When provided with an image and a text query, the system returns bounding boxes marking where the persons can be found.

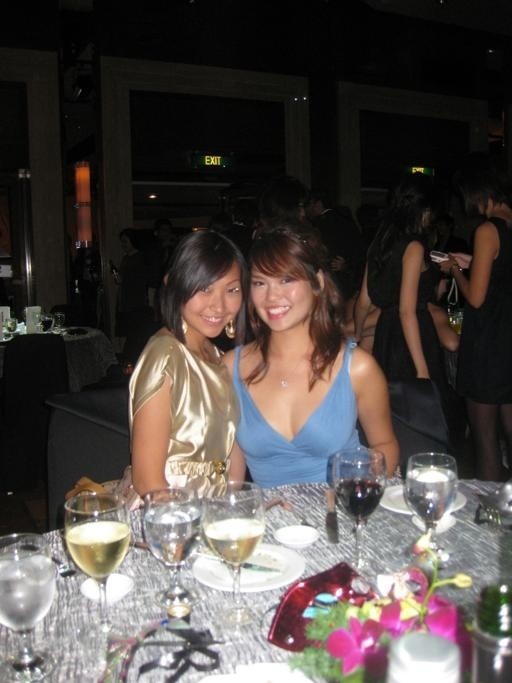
[127,229,247,505]
[223,215,401,491]
[352,174,456,477]
[435,167,512,483]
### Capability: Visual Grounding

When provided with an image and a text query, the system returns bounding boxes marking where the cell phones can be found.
[430,250,449,264]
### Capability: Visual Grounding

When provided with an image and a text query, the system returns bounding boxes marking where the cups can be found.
[0,306,66,337]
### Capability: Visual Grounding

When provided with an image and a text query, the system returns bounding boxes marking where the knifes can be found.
[324,487,339,545]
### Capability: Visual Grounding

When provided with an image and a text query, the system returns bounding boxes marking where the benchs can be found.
[44,376,455,528]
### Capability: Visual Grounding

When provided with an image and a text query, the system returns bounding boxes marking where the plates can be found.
[382,482,469,517]
[271,525,320,550]
[191,545,307,591]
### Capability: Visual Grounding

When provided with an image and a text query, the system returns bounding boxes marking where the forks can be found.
[475,489,503,533]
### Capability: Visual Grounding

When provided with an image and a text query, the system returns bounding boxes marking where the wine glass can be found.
[402,450,459,574]
[63,491,145,650]
[330,445,390,575]
[203,479,271,631]
[0,529,65,683]
[137,488,206,611]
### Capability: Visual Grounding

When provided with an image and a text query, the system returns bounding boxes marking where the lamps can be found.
[74,161,94,247]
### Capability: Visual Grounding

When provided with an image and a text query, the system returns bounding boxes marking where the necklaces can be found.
[264,358,308,387]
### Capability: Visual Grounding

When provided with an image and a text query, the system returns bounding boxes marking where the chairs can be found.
[1,332,71,491]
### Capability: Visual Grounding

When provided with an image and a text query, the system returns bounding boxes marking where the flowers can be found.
[298,530,474,683]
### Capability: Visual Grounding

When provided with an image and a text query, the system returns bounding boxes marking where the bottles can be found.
[381,632,463,683]
[473,585,512,682]
